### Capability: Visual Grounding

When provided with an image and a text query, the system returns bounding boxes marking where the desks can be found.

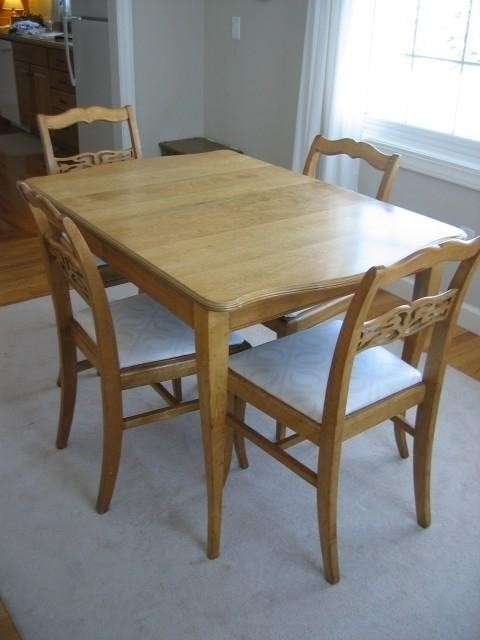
[22,150,468,560]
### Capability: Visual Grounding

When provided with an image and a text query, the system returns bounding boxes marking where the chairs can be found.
[35,104,147,393]
[224,238,479,585]
[260,134,403,444]
[16,181,249,515]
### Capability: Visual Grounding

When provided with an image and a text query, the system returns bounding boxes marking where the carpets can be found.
[2,280,479,640]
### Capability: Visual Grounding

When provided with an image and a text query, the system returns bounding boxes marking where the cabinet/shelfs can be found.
[157,138,244,159]
[12,42,77,146]
